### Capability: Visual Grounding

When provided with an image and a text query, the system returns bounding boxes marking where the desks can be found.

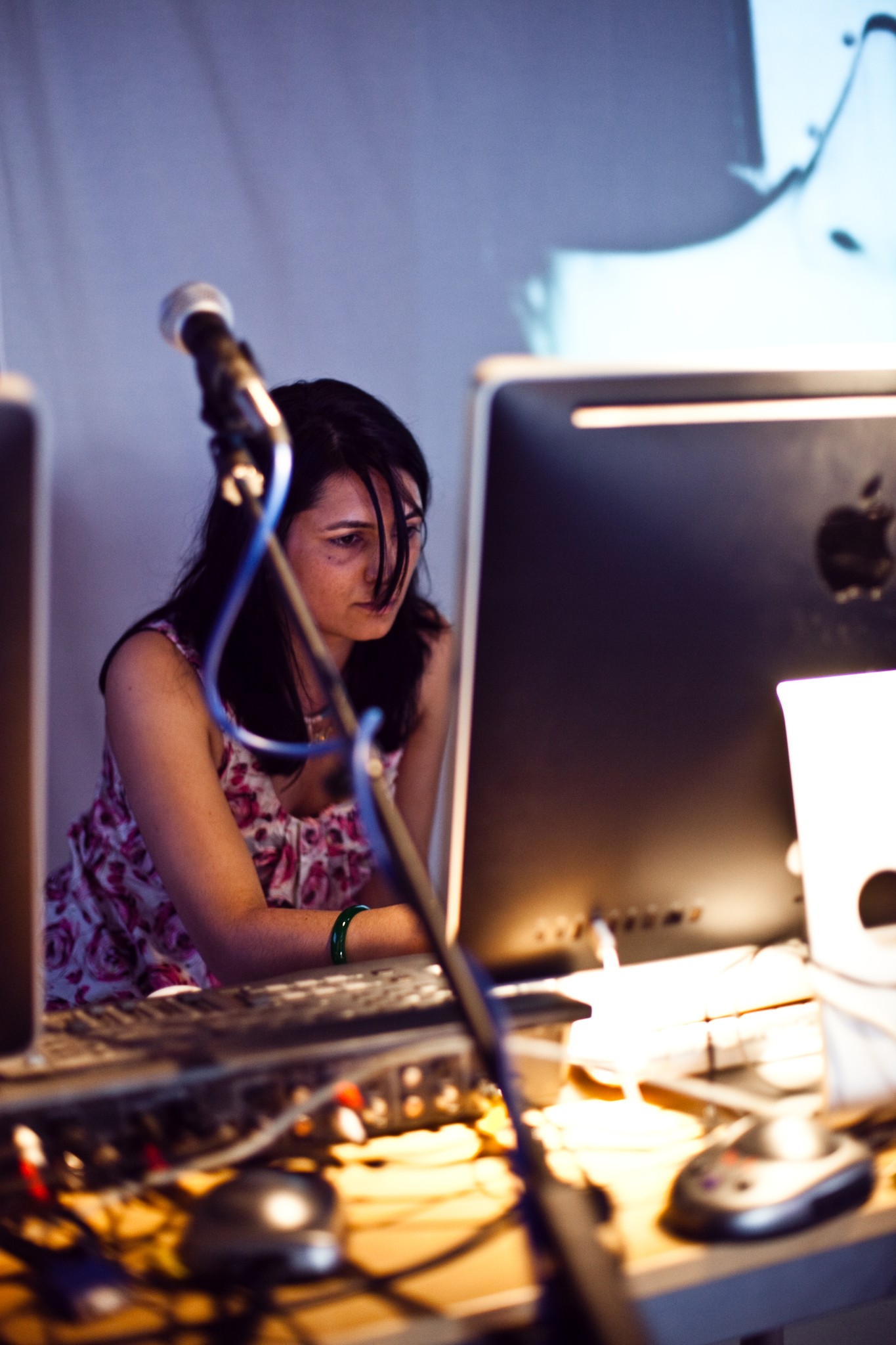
[0,954,896,1345]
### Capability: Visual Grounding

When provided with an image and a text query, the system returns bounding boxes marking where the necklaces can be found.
[303,708,336,743]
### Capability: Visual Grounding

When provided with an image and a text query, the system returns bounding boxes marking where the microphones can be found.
[161,283,285,421]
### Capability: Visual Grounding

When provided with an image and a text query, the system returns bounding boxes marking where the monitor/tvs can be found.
[445,365,894,980]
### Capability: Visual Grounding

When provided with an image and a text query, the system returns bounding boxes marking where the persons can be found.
[41,378,457,1011]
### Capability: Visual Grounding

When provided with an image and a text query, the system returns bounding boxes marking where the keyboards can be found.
[67,943,477,1060]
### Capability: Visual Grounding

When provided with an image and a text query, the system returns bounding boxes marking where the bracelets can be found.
[331,905,371,965]
